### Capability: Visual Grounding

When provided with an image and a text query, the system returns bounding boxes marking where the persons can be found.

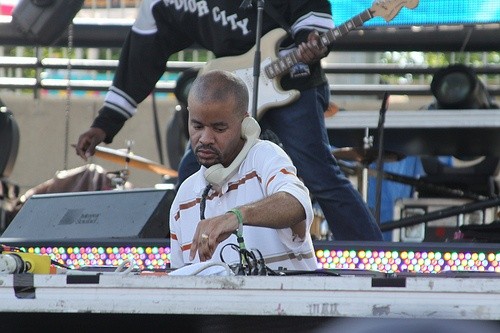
[169,71,317,269]
[77,0,384,242]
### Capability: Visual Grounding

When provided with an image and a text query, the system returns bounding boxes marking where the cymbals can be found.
[70,144,178,177]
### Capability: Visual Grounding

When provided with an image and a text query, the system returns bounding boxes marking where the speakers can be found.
[0,189,176,244]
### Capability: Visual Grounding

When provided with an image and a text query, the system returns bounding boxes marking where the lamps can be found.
[419,63,500,188]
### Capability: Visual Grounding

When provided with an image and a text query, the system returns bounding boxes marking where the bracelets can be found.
[225,209,248,266]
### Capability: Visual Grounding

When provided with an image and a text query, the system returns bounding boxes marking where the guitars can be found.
[196,0,420,117]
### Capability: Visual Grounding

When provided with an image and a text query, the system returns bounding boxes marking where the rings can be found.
[201,234,209,239]
